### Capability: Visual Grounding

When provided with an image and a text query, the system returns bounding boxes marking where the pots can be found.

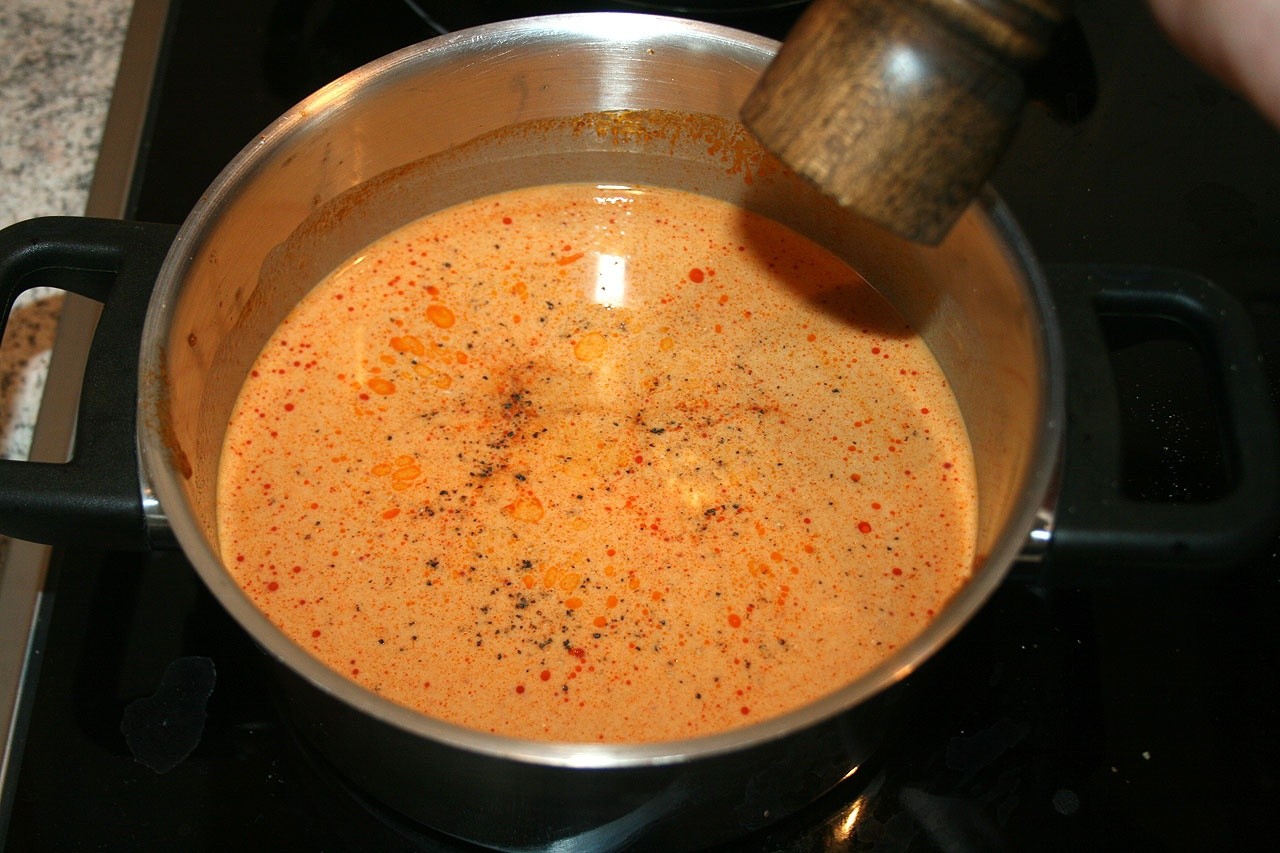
[0,10,1280,770]
